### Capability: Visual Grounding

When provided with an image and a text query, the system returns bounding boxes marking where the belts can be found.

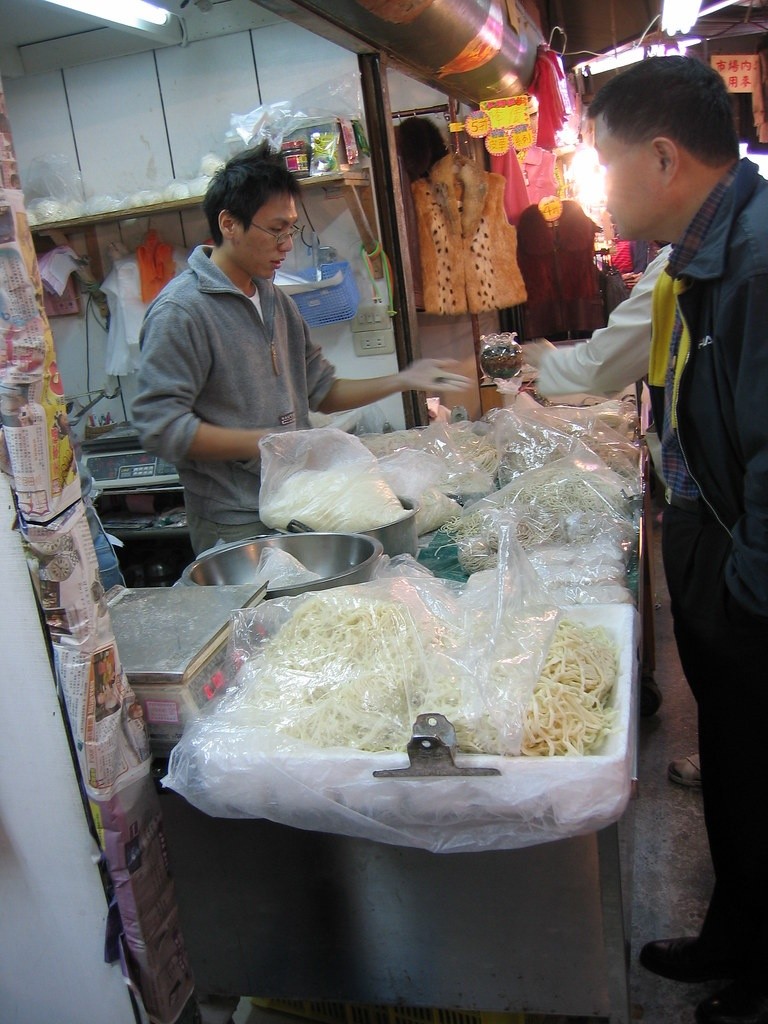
[665,487,701,515]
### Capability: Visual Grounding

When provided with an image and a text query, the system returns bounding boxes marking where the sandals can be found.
[668,754,702,785]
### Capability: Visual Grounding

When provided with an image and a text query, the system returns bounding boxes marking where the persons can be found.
[128,142,477,556]
[517,54,768,1023]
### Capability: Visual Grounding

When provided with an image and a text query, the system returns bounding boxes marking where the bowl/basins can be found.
[272,497,419,565]
[182,532,385,600]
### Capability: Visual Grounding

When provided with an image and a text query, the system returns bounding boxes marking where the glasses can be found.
[246,220,301,244]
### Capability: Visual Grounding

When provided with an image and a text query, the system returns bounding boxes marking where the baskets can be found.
[291,261,360,327]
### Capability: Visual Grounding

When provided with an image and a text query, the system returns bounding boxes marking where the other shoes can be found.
[638,936,701,984]
